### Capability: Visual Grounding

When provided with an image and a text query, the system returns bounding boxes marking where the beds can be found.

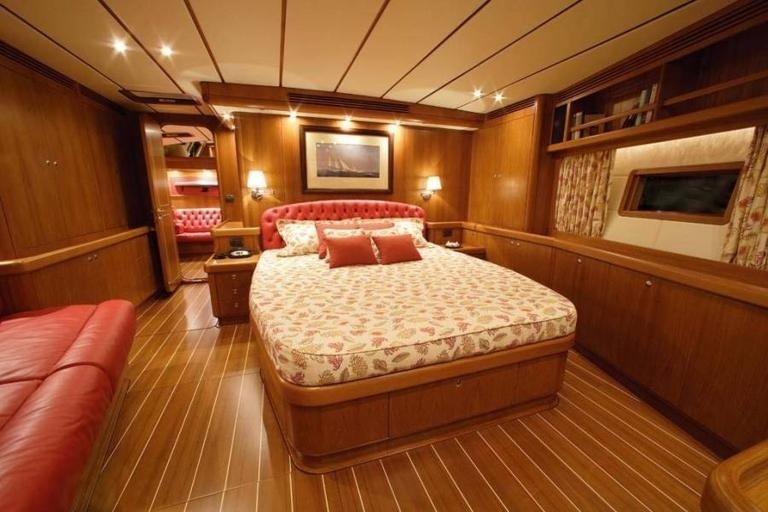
[249,199,578,475]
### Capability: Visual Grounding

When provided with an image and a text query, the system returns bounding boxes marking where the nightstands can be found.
[203,250,261,327]
[435,242,486,260]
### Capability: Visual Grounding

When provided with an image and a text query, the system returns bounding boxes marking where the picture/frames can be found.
[299,124,394,196]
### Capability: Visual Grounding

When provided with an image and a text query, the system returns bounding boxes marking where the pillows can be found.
[276,217,429,269]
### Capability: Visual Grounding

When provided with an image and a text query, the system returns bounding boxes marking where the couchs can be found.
[1,299,137,511]
[173,208,222,256]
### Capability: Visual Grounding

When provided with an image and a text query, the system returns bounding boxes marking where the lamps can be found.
[247,170,267,201]
[420,175,442,201]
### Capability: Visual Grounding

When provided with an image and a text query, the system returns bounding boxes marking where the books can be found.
[571,83,658,139]
